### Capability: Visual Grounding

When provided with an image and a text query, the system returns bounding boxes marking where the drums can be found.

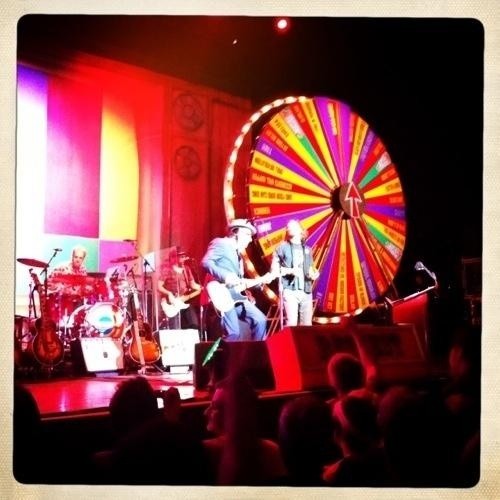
[71,302,126,338]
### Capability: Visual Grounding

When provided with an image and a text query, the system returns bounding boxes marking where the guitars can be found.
[128,288,160,365]
[28,268,64,366]
[206,268,293,313]
[161,289,202,318]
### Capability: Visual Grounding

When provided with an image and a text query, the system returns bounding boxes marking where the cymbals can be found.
[17,258,49,267]
[110,256,137,262]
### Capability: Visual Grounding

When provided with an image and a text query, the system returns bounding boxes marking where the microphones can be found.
[54,248,63,252]
[301,236,305,250]
[126,262,138,277]
[123,239,138,242]
[410,259,437,284]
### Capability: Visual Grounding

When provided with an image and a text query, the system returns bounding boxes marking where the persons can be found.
[49,247,88,276]
[201,219,268,344]
[157,247,204,329]
[14,333,481,488]
[270,220,320,326]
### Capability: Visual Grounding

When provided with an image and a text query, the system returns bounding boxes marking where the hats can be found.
[227,218,257,236]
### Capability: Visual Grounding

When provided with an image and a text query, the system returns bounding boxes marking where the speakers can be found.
[193,340,276,391]
[348,322,439,385]
[263,325,367,393]
